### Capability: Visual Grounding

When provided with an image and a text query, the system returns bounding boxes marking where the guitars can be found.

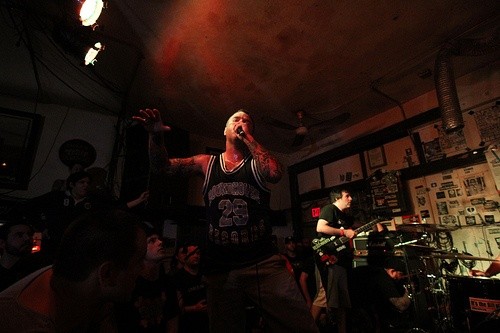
[312,212,385,259]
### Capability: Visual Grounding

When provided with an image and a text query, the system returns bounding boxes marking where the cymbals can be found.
[429,254,498,260]
[394,220,462,230]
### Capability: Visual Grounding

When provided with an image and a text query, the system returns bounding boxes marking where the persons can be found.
[272,186,415,333]
[132,108,320,333]
[469,255,500,277]
[0,167,210,333]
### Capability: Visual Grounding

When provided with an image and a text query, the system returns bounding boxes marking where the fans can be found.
[261,109,350,147]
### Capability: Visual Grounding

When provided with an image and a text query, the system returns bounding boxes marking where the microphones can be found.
[236,126,245,136]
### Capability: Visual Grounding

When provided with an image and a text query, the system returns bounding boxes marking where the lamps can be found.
[69,0,108,31]
[69,39,105,66]
